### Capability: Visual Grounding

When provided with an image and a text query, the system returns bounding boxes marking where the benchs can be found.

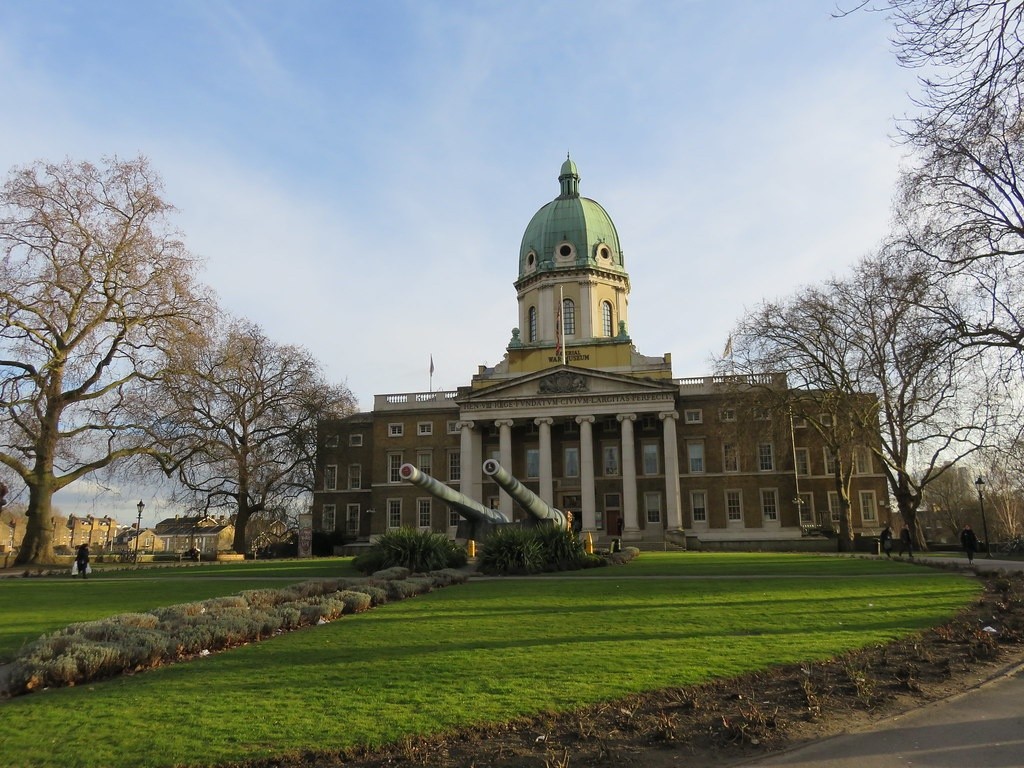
[179,554,201,563]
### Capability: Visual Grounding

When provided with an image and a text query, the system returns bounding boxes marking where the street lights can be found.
[975,477,994,560]
[133,499,146,564]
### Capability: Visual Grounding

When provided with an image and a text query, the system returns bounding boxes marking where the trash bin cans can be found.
[612,538,622,552]
[872,538,881,555]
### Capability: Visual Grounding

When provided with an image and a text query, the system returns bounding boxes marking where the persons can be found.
[72,543,89,579]
[899,524,915,557]
[880,526,893,557]
[190,545,201,563]
[960,524,982,565]
[616,514,623,536]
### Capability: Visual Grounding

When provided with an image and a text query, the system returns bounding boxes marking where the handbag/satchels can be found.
[71,561,78,575]
[884,539,892,549]
[86,563,92,574]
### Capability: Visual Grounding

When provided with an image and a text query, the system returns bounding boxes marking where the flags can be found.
[555,302,562,353]
